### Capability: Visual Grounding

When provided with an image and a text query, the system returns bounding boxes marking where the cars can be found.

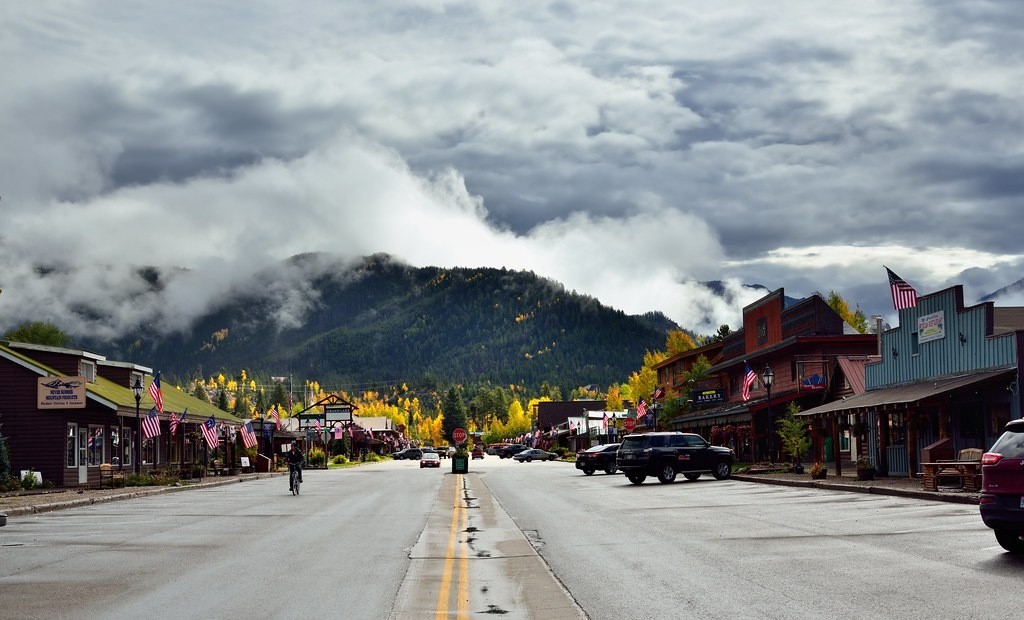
[420,453,442,468]
[513,449,558,463]
[576,443,621,475]
[471,447,484,460]
[500,444,528,458]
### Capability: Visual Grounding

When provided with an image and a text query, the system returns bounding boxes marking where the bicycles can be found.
[287,462,302,495]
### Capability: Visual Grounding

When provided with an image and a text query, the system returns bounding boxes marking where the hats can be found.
[291,441,296,446]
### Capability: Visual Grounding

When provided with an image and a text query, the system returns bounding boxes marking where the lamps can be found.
[958,332,966,347]
[892,347,898,359]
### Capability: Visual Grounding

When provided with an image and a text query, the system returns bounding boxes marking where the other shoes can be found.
[300,480,303,483]
[289,488,292,491]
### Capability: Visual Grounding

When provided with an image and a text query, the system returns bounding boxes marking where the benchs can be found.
[99,463,124,488]
[213,459,229,477]
[935,448,984,486]
[916,472,983,479]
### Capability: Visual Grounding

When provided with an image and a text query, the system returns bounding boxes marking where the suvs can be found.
[979,417,1024,550]
[393,447,455,460]
[615,431,735,484]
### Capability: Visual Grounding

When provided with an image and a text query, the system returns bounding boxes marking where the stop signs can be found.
[452,428,467,443]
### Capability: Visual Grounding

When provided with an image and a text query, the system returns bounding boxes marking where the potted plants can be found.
[854,455,876,481]
[809,461,828,479]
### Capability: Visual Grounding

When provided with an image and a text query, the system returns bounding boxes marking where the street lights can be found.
[555,426,558,449]
[363,429,367,461]
[651,399,658,432]
[259,408,265,454]
[612,413,617,442]
[305,418,311,465]
[577,422,581,451]
[132,378,143,474]
[761,363,774,465]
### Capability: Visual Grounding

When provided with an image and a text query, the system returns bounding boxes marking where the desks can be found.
[922,461,980,492]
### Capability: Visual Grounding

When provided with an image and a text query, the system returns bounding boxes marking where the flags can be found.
[368,428,374,439]
[502,430,542,448]
[570,421,575,434]
[202,414,236,449]
[169,407,188,436]
[333,426,354,440]
[142,407,161,437]
[741,364,757,401]
[314,416,322,431]
[148,373,165,412]
[886,268,918,311]
[270,383,293,431]
[240,422,258,450]
[636,396,647,420]
[381,433,421,450]
[87,427,101,447]
[551,424,555,434]
[603,413,608,430]
[655,386,661,398]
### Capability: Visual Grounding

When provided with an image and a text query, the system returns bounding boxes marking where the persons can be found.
[284,442,305,491]
